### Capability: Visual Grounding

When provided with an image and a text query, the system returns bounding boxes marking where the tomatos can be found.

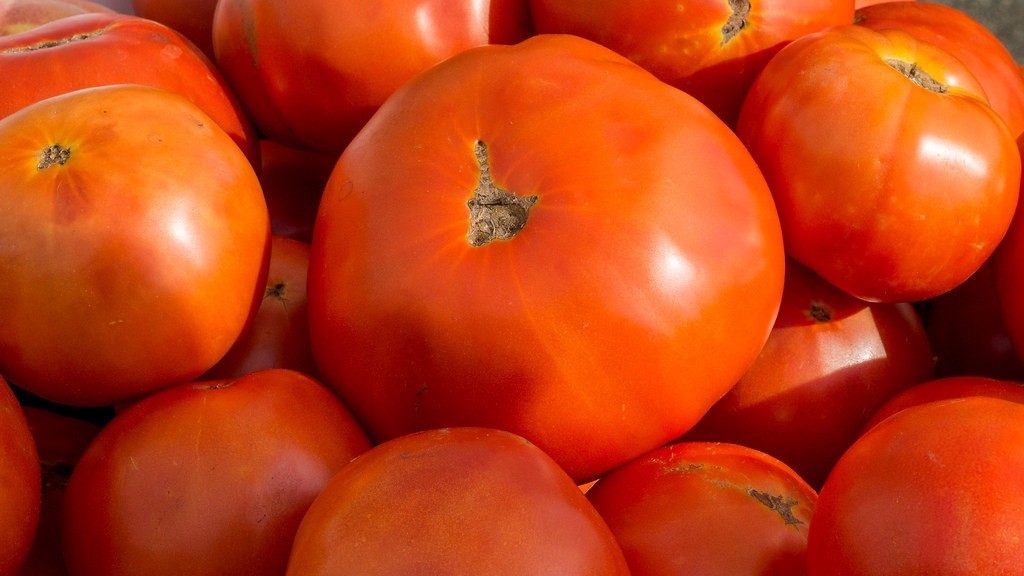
[0,0,1024,576]
